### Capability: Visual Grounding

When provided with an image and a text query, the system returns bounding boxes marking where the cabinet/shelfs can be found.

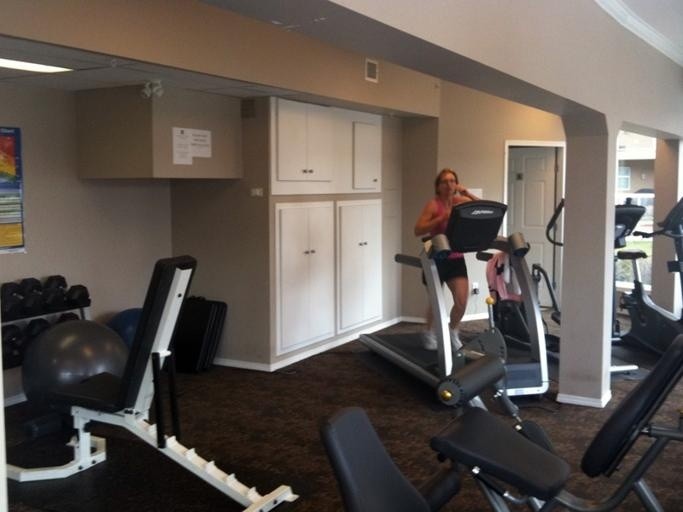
[171,95,383,375]
[0,305,91,408]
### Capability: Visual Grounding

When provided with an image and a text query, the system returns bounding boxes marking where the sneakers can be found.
[450,326,463,350]
[422,329,438,351]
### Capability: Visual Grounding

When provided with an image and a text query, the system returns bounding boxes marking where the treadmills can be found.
[474,247,640,375]
[358,201,549,402]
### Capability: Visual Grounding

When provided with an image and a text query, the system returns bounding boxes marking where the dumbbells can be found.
[0,276,90,368]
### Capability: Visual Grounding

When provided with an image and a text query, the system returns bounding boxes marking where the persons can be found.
[414,168,481,353]
